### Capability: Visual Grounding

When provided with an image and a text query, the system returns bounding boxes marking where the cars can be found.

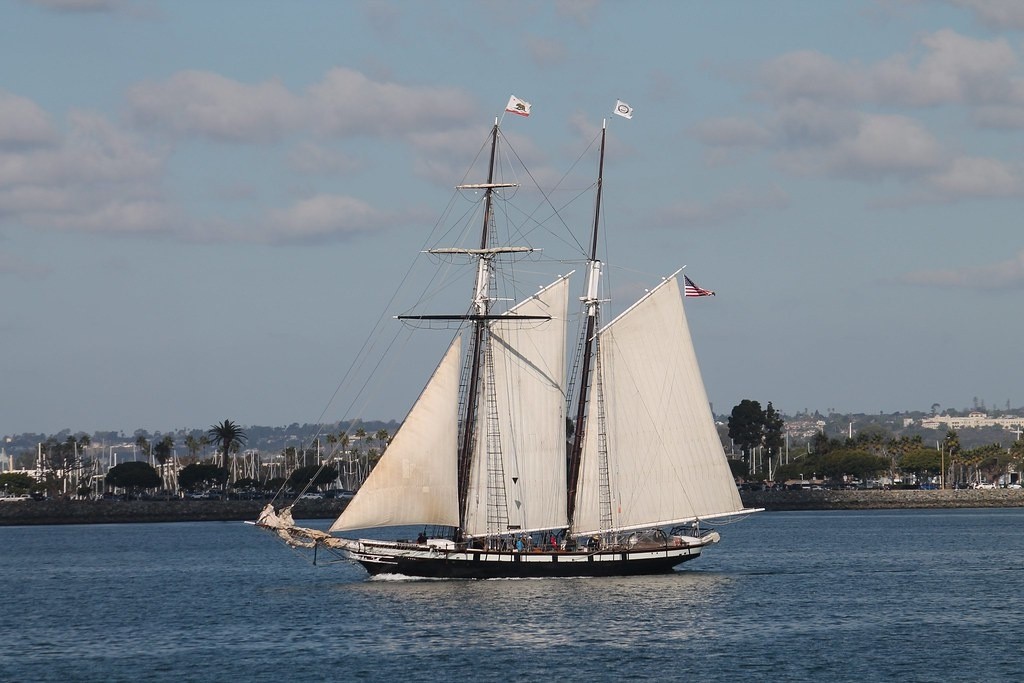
[0,486,356,504]
[734,481,1023,491]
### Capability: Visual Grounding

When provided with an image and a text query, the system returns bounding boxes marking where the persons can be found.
[497,530,601,553]
[416,532,429,544]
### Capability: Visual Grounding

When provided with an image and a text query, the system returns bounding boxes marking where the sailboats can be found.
[244,94,767,577]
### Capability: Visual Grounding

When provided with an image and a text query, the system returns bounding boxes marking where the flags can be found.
[613,99,634,120]
[505,94,533,118]
[684,273,715,298]
